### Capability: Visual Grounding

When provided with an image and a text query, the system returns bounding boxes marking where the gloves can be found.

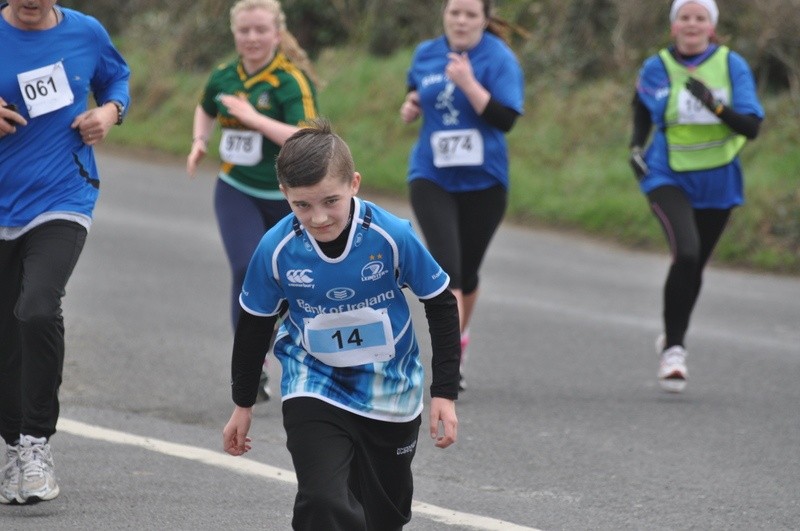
[685,77,722,114]
[630,145,648,181]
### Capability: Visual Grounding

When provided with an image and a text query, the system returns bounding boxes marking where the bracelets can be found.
[102,99,126,125]
[711,99,722,112]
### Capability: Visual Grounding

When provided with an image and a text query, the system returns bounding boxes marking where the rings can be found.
[88,133,92,141]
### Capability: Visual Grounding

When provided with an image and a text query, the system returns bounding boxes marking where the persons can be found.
[629,0,765,393]
[0,0,132,506]
[398,1,531,391]
[184,1,326,403]
[221,117,461,530]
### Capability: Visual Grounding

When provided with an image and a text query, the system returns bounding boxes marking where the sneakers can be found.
[1,445,27,505]
[658,344,688,392]
[16,434,60,504]
